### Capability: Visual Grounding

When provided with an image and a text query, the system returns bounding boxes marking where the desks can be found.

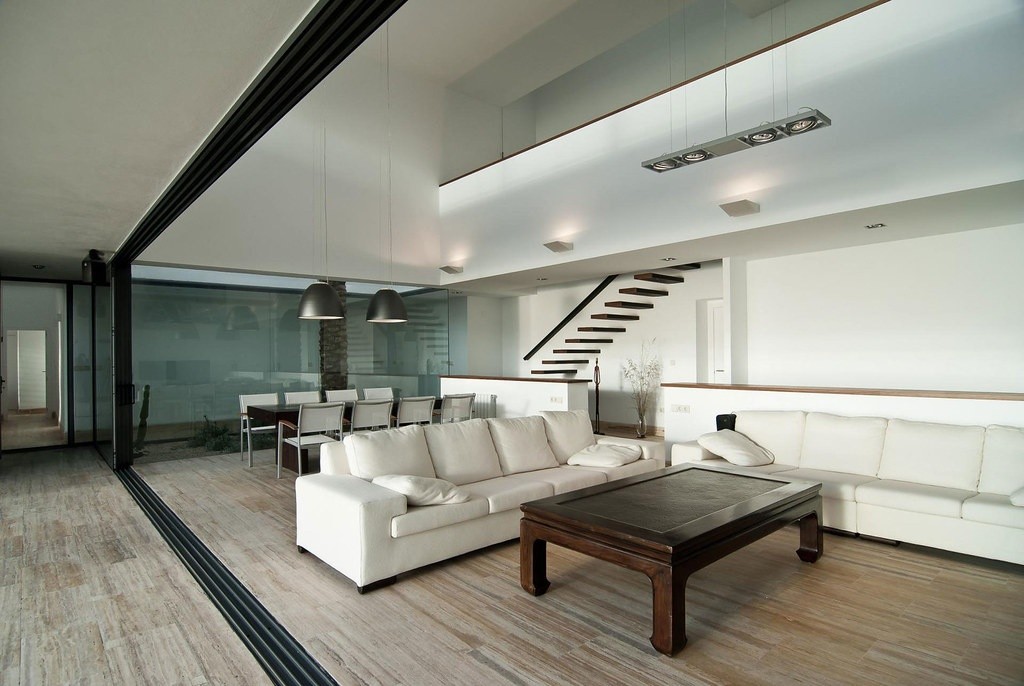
[248,398,443,474]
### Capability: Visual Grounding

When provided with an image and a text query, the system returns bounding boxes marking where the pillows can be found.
[1010,488,1024,506]
[697,429,775,466]
[567,444,643,468]
[371,474,472,506]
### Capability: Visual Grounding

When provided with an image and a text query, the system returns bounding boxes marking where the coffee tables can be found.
[519,462,823,655]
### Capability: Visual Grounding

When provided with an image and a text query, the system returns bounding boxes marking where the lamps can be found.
[439,266,463,275]
[297,81,345,320]
[719,199,760,217]
[543,242,573,253]
[175,306,314,339]
[641,0,831,173]
[365,19,409,323]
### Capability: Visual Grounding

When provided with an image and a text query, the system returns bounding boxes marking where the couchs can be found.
[671,410,1024,567]
[295,410,666,595]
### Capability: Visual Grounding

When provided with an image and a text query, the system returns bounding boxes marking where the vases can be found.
[637,417,647,439]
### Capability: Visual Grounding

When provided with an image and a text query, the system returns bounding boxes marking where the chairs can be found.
[239,387,476,479]
[149,382,310,434]
